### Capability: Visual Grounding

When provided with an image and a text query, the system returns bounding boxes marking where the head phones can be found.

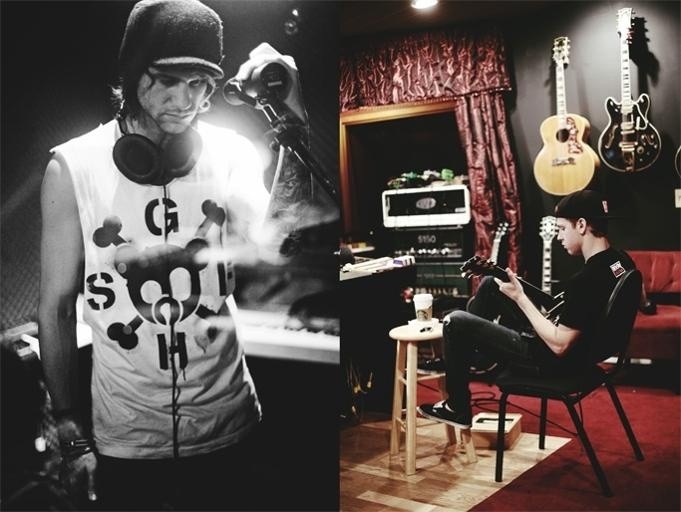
[113,114,205,184]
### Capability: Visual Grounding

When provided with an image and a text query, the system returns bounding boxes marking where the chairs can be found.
[494,268,644,496]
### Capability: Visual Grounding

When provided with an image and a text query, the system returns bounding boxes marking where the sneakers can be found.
[415,397,473,430]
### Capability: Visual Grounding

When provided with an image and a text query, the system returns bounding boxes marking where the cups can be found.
[413,293,433,322]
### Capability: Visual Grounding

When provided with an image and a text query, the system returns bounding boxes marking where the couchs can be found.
[606,249,681,376]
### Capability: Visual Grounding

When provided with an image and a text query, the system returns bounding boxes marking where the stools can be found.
[388,319,477,473]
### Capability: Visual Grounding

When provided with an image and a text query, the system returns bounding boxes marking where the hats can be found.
[554,189,627,221]
[115,0,226,82]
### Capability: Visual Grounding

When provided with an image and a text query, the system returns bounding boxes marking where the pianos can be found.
[238,302,340,365]
[339,255,415,281]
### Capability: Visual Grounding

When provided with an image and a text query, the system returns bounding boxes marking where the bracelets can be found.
[48,406,79,422]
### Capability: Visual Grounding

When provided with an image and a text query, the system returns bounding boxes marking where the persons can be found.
[414,187,648,430]
[33,0,314,512]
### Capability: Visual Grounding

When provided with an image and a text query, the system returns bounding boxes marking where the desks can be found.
[342,252,418,426]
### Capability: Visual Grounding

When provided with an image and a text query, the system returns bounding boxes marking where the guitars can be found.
[597,7,661,173]
[458,215,564,326]
[532,36,601,196]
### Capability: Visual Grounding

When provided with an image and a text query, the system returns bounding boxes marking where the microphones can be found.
[221,60,292,106]
